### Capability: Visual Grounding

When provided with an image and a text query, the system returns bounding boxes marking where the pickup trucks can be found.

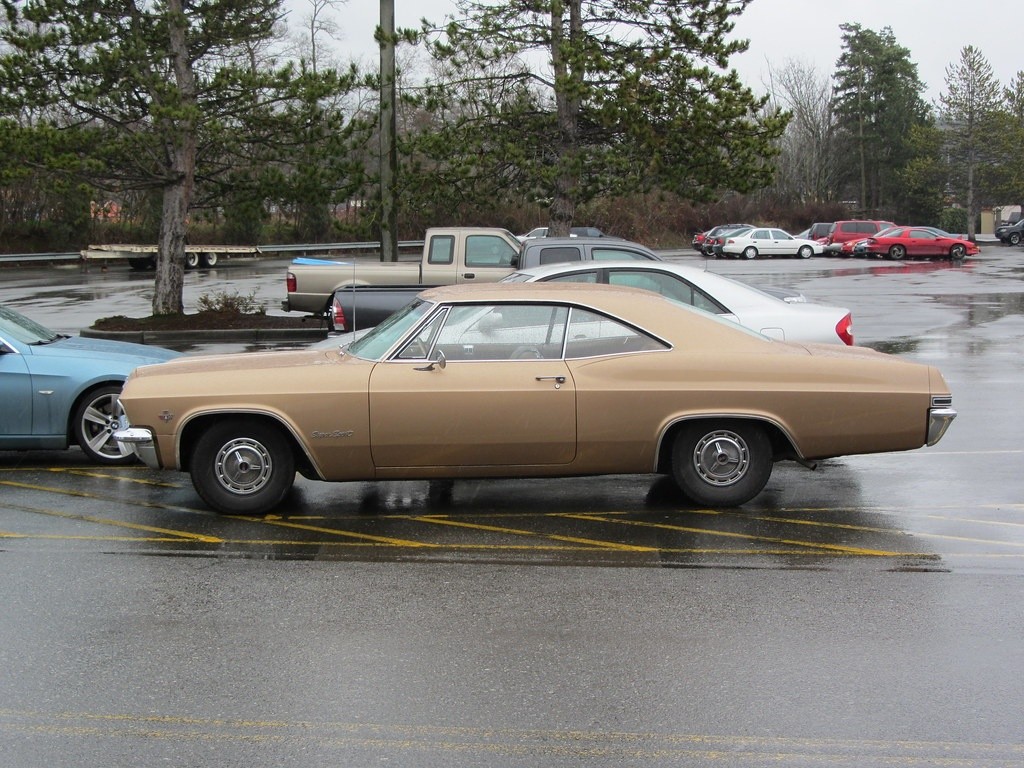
[280,226,523,318]
[320,236,668,339]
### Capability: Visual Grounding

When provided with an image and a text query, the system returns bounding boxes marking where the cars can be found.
[108,281,960,518]
[0,304,188,469]
[721,227,825,261]
[793,219,966,258]
[305,260,856,362]
[570,225,604,238]
[863,226,981,261]
[516,227,579,245]
[691,221,758,259]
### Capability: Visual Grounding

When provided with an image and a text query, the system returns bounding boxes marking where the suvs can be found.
[995,217,1024,246]
